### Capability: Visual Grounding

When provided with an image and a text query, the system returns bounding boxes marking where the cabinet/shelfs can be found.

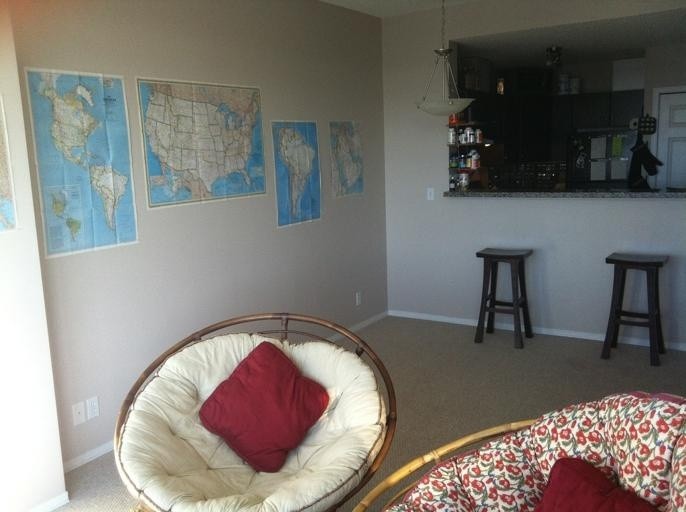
[449,89,644,190]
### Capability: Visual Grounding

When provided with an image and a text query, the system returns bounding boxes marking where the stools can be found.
[601,253,669,366]
[475,248,533,349]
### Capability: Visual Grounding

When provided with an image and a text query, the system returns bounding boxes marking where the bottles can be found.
[448,127,484,145]
[450,176,456,191]
[452,150,481,170]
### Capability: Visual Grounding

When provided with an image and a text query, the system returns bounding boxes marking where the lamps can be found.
[416,0,476,116]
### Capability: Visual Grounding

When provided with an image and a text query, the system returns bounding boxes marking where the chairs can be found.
[114,313,397,512]
[352,416,686,512]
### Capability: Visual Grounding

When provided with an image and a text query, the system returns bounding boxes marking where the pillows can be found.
[534,458,661,512]
[200,341,329,473]
